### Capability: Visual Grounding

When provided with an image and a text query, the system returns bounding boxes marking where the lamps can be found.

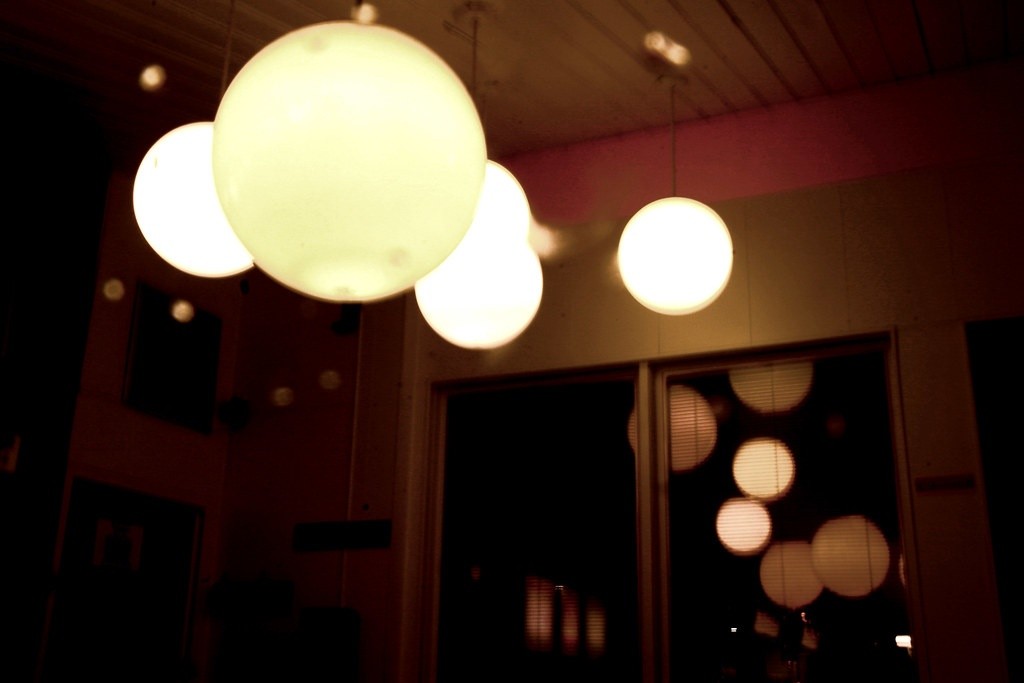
[618,74,732,317]
[129,3,546,351]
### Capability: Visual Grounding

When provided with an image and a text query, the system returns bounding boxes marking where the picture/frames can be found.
[117,273,223,436]
